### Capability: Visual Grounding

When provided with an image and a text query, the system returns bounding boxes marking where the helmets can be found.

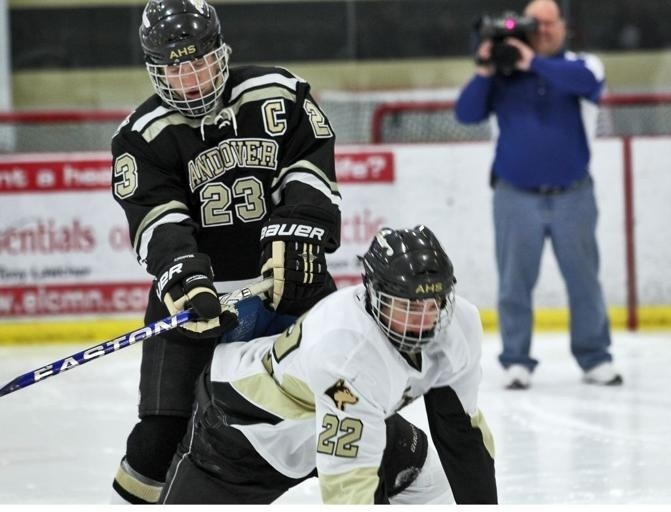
[361,225,456,353]
[138,0,232,118]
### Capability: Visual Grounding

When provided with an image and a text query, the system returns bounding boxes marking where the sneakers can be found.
[502,363,530,388]
[583,363,622,384]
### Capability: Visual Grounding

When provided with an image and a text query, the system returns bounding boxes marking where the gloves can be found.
[154,251,239,340]
[259,218,329,314]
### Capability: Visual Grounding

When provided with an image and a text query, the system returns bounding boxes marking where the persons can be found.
[105,0,346,504]
[448,0,628,391]
[149,221,502,502]
